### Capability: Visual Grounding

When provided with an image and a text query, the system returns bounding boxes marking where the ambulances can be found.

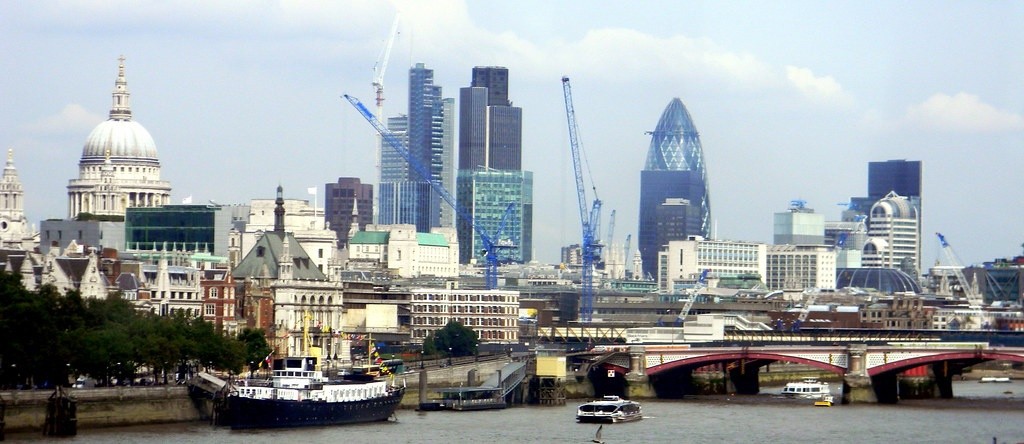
[72,378,95,389]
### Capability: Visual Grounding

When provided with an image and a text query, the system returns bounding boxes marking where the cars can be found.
[732,285,1015,309]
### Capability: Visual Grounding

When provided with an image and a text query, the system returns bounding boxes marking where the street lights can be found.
[448,347,452,365]
[391,354,395,375]
[421,349,425,369]
[475,343,478,361]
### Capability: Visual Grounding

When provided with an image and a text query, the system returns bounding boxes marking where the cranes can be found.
[561,76,607,322]
[341,93,517,291]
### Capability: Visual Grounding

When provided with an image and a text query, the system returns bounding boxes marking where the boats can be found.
[978,375,1010,383]
[415,382,508,411]
[812,394,835,407]
[189,312,405,428]
[574,394,643,424]
[780,380,830,400]
